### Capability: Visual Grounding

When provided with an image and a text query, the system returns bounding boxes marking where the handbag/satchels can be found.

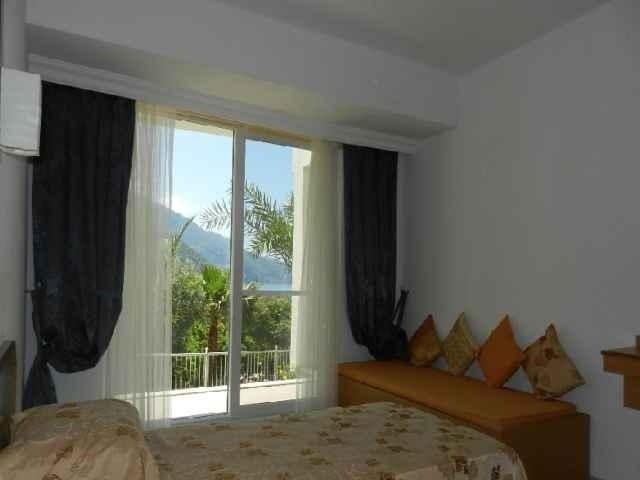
[367,325,409,362]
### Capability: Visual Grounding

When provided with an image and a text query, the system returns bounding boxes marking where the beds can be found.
[1,336,529,480]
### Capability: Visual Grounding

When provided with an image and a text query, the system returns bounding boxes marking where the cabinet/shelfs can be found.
[339,358,577,480]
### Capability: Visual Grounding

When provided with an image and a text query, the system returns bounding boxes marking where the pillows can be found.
[407,315,443,365]
[475,314,528,386]
[440,311,480,377]
[522,323,586,400]
